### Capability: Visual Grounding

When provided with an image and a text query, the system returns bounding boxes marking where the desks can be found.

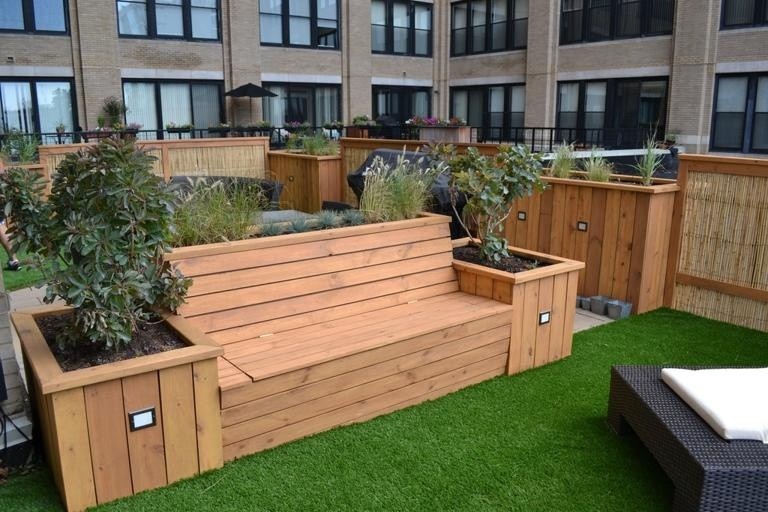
[603,351,767,510]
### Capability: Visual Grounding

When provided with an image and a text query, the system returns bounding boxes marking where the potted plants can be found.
[472,125,682,317]
[432,136,588,374]
[55,123,66,133]
[264,130,344,208]
[346,114,377,141]
[8,130,227,511]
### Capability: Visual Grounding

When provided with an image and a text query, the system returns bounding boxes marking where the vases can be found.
[576,292,633,320]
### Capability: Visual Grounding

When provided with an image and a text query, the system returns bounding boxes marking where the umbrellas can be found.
[223,83,279,122]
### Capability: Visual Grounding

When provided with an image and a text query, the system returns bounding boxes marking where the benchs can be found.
[155,209,518,467]
